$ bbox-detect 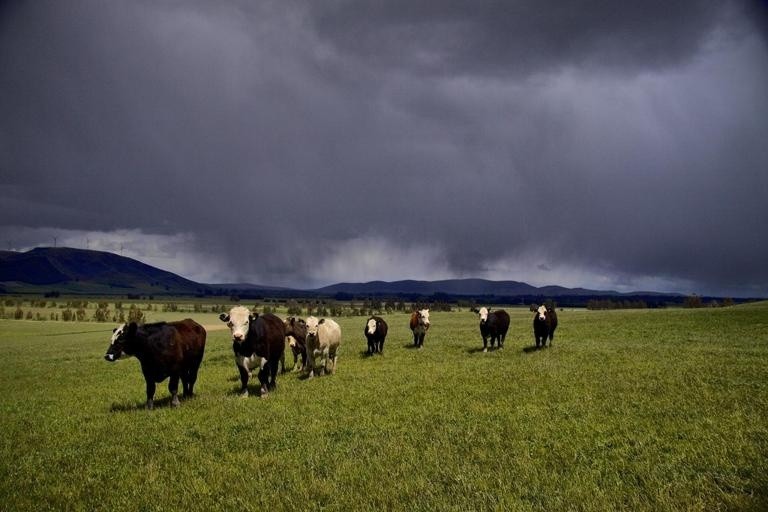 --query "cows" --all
[365,315,388,356]
[219,305,341,400]
[474,306,510,352]
[410,306,431,348]
[533,304,558,349]
[104,318,206,410]
